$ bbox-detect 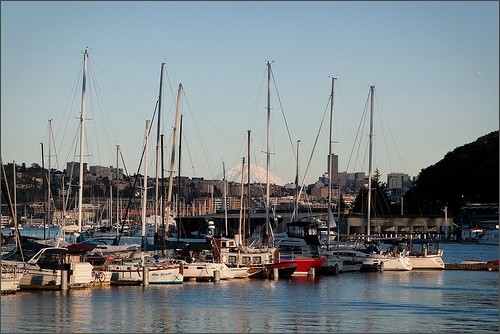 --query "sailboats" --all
[0,44,445,292]
